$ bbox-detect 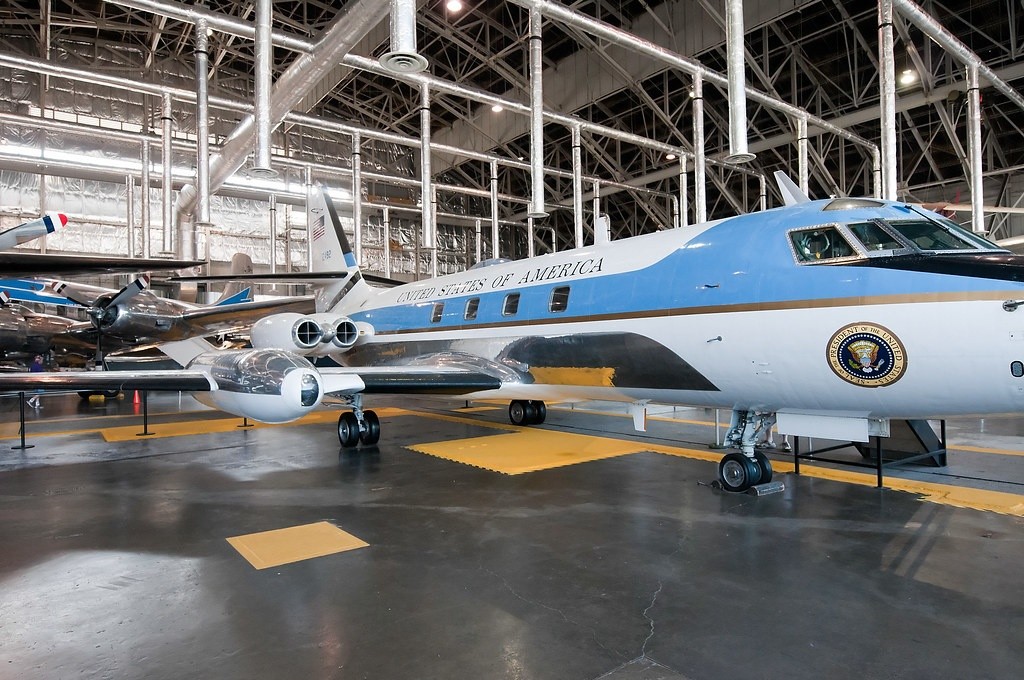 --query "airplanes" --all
[1,183,1024,495]
